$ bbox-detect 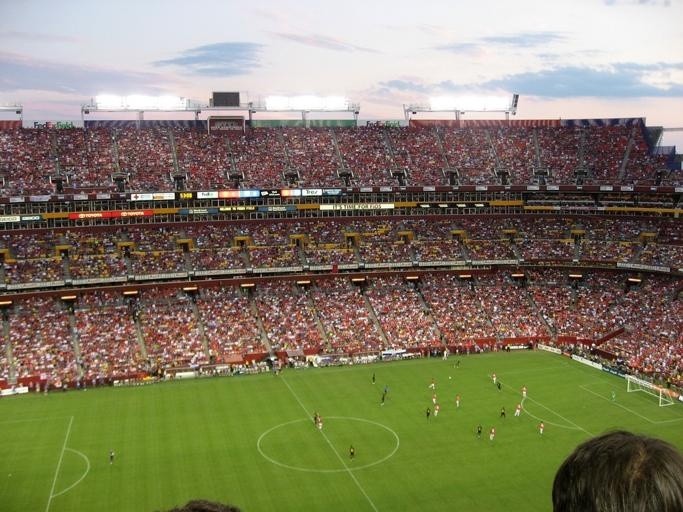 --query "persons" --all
[314,412,322,429]
[109,451,114,464]
[1,217,682,295]
[380,371,544,440]
[553,432,682,511]
[2,125,682,198]
[350,445,354,457]
[1,272,682,395]
